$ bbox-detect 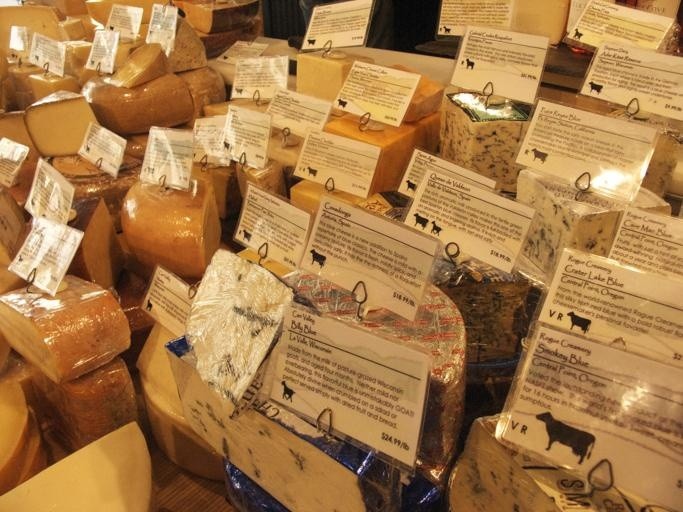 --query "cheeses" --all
[0,0,683,512]
